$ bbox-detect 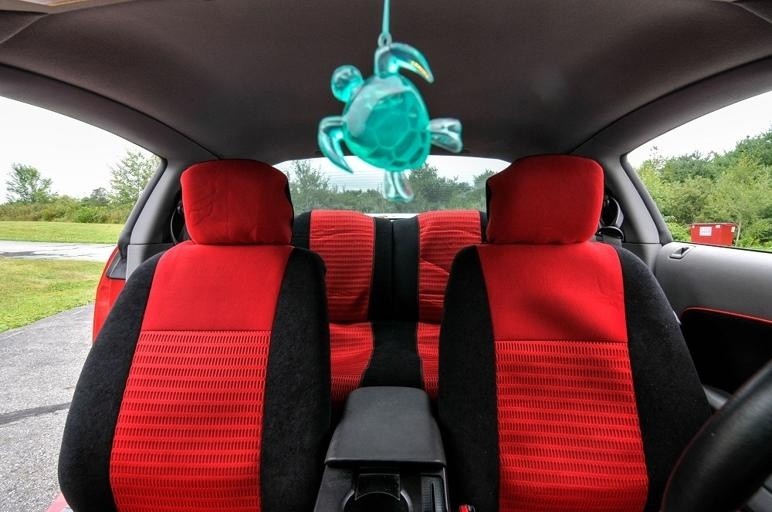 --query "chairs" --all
[293,210,394,401]
[393,209,488,397]
[57,160,333,512]
[436,153,712,512]
